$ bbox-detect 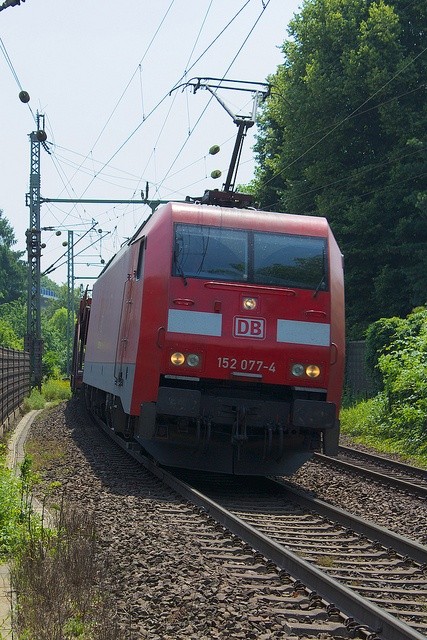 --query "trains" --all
[69,201,346,476]
